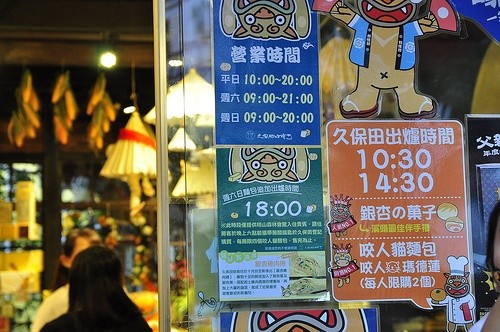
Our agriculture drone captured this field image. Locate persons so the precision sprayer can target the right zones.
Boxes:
[466,202,500,331]
[30,227,129,332]
[38,246,153,332]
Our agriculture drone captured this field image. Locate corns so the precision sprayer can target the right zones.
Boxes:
[7,70,116,150]
